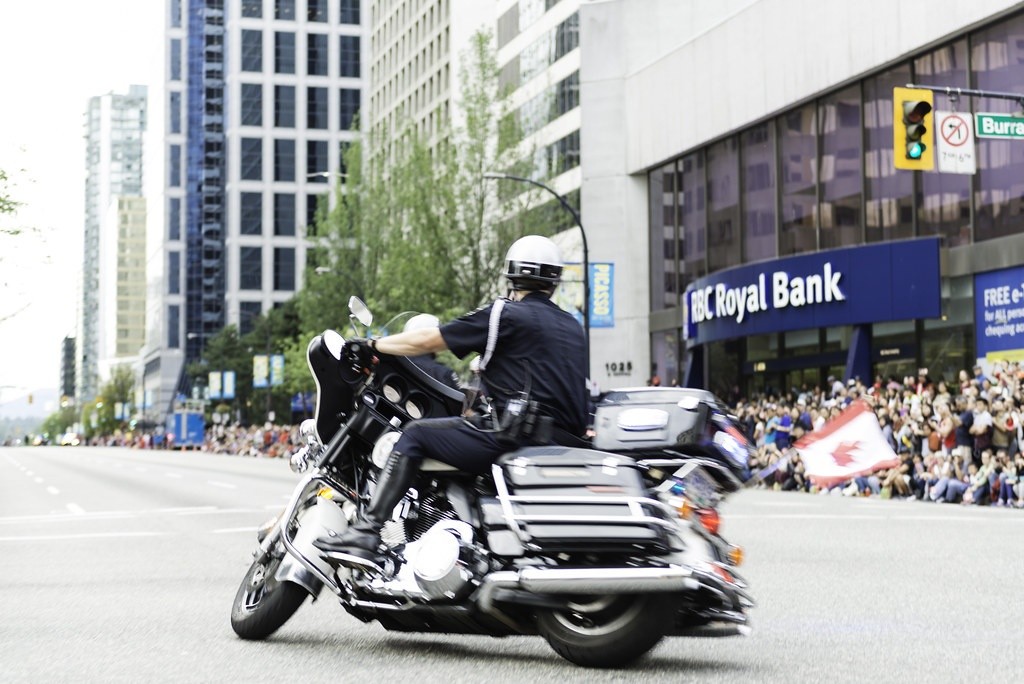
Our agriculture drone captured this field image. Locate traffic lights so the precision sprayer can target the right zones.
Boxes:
[893,86,935,170]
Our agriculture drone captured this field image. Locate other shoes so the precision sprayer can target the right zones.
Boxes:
[830,486,841,495]
[907,495,1024,509]
[819,487,830,495]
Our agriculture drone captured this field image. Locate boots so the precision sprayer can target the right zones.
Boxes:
[312,443,424,560]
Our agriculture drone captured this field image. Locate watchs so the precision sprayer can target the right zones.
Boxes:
[370,335,384,355]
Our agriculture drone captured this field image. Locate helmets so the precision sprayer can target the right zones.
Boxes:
[501,234,565,286]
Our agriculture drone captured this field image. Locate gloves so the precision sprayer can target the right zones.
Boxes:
[340,337,375,377]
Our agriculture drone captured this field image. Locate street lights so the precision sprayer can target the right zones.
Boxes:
[484,168,590,383]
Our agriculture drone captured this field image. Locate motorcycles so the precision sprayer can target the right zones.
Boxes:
[229,296,759,671]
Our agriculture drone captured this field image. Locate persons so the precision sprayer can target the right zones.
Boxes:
[650,376,662,386]
[312,234,590,561]
[672,378,681,387]
[714,359,1024,510]
[90,421,301,458]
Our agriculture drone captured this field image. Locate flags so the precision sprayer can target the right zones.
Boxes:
[791,398,900,492]
